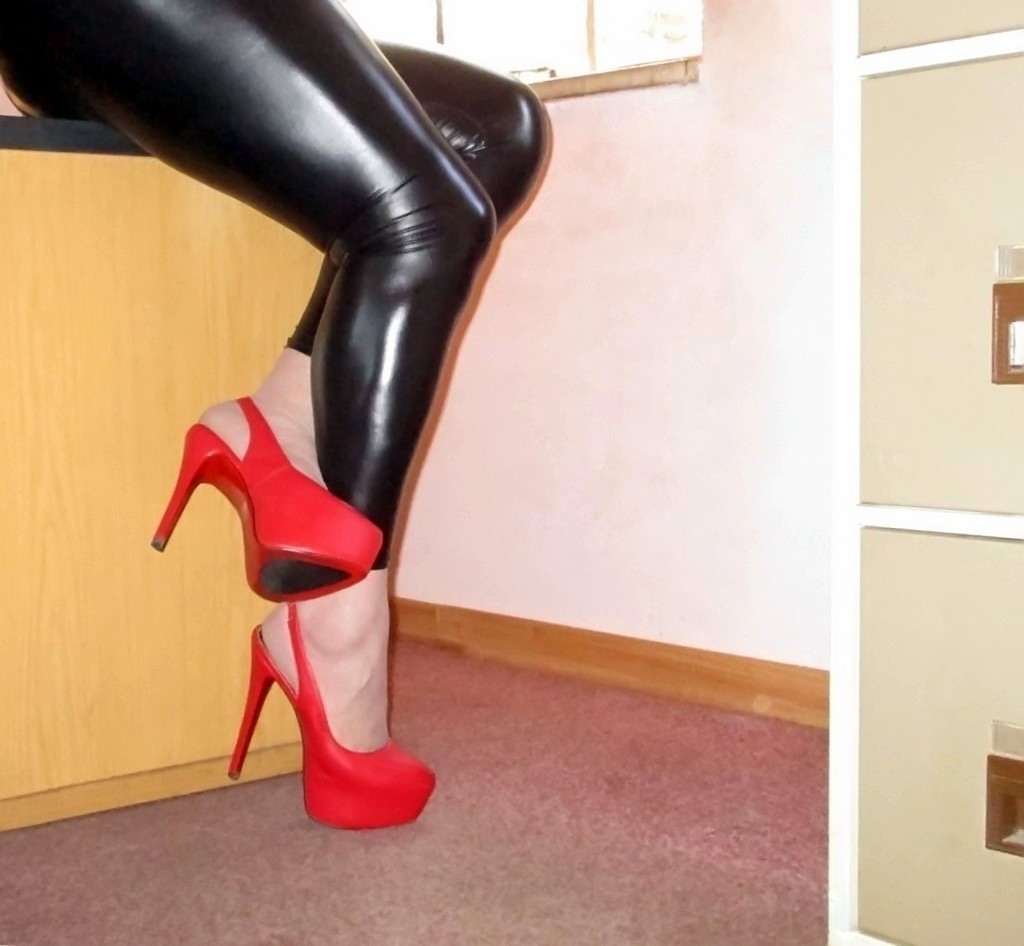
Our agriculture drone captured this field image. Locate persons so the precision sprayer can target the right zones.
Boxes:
[0,0,554,829]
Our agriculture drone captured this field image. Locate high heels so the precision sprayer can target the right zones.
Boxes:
[227,603,438,829]
[151,397,382,603]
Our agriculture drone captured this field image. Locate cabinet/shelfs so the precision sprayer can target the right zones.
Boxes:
[0,115,324,831]
